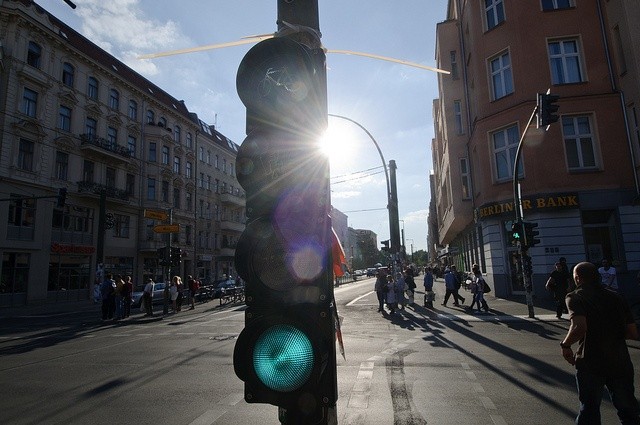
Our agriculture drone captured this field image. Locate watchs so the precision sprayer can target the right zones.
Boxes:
[561,341,571,349]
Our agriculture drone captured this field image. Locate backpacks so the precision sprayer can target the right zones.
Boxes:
[120,284,128,297]
[193,280,200,290]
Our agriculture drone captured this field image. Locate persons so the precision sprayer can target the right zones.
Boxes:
[561,262,640,418]
[100,273,133,320]
[599,259,618,289]
[545,262,567,320]
[451,265,461,306]
[143,278,155,317]
[473,264,482,279]
[474,271,490,314]
[558,257,570,314]
[170,276,183,314]
[187,275,199,310]
[466,268,483,314]
[219,273,242,289]
[441,269,465,305]
[373,263,417,315]
[423,267,434,309]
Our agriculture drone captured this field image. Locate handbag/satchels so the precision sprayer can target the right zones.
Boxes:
[483,279,491,292]
[403,283,409,292]
[143,283,153,297]
[425,289,436,302]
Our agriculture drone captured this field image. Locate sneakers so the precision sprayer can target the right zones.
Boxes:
[462,298,465,304]
[453,302,455,304]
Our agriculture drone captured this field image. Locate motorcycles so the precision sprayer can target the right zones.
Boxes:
[380,267,388,278]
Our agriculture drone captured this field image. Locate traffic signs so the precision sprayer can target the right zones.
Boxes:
[144,209,169,221]
[152,225,180,233]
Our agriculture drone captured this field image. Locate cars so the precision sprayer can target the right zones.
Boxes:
[130,283,179,308]
[363,270,368,274]
[356,270,362,275]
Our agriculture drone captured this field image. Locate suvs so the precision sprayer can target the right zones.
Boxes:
[367,268,376,277]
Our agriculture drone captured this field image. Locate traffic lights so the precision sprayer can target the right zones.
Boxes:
[172,248,181,265]
[512,223,523,242]
[525,223,540,248]
[57,188,68,207]
[537,92,560,130]
[233,26,333,422]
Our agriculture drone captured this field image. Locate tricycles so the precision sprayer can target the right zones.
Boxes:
[220,286,244,305]
